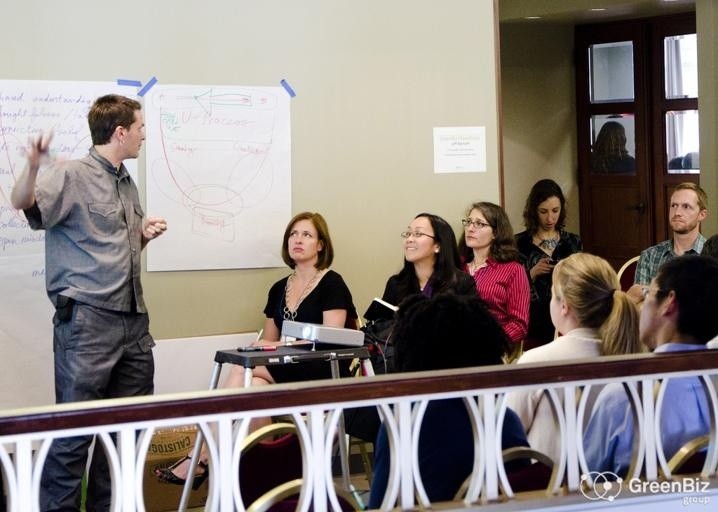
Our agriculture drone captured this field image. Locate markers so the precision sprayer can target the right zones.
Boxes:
[237,345,277,352]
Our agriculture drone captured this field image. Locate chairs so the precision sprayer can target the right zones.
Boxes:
[451,446,570,503]
[251,306,375,487]
[234,421,305,508]
[240,477,361,512]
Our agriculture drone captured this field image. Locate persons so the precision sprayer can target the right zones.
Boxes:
[359,178,718,512]
[594,120,637,173]
[151,213,359,490]
[11,95,169,511]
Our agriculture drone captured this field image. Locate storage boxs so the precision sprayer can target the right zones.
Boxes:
[134,424,210,512]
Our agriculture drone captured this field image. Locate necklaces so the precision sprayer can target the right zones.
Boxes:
[283,268,323,319]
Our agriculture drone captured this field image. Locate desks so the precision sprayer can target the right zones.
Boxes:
[175,342,391,512]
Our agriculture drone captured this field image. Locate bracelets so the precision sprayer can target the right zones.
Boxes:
[285,340,293,347]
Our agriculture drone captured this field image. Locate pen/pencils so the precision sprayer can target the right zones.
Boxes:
[256,328,264,341]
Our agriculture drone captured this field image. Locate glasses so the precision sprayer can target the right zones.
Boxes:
[461,219,490,229]
[400,231,434,240]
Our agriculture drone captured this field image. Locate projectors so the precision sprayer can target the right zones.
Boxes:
[282,320,365,351]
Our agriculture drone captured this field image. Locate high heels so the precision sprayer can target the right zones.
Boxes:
[154,456,208,490]
